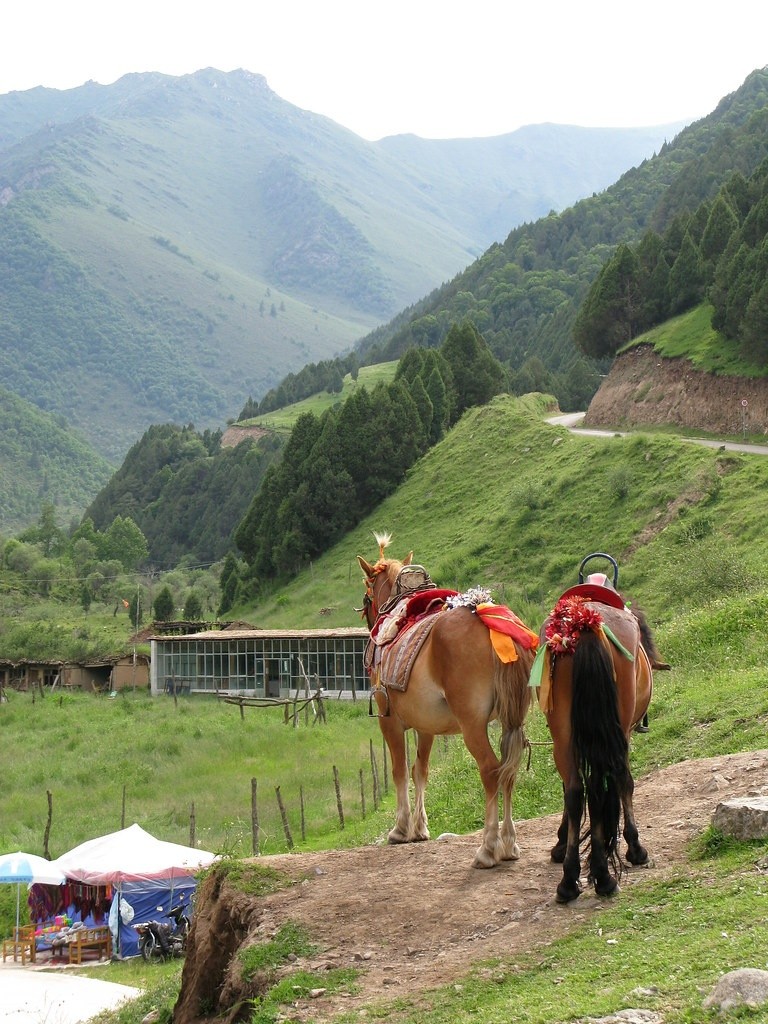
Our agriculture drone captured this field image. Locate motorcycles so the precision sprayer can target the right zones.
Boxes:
[130,893,192,967]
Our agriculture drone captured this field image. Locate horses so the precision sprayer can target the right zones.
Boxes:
[355,531,541,871]
[536,571,671,905]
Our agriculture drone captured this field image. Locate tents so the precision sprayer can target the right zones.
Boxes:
[36,821,229,961]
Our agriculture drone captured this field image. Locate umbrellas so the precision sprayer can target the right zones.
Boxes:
[0,850,67,943]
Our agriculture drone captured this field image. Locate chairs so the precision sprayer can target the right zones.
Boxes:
[2,926,36,965]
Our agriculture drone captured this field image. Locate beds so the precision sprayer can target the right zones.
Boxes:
[21,919,110,965]
